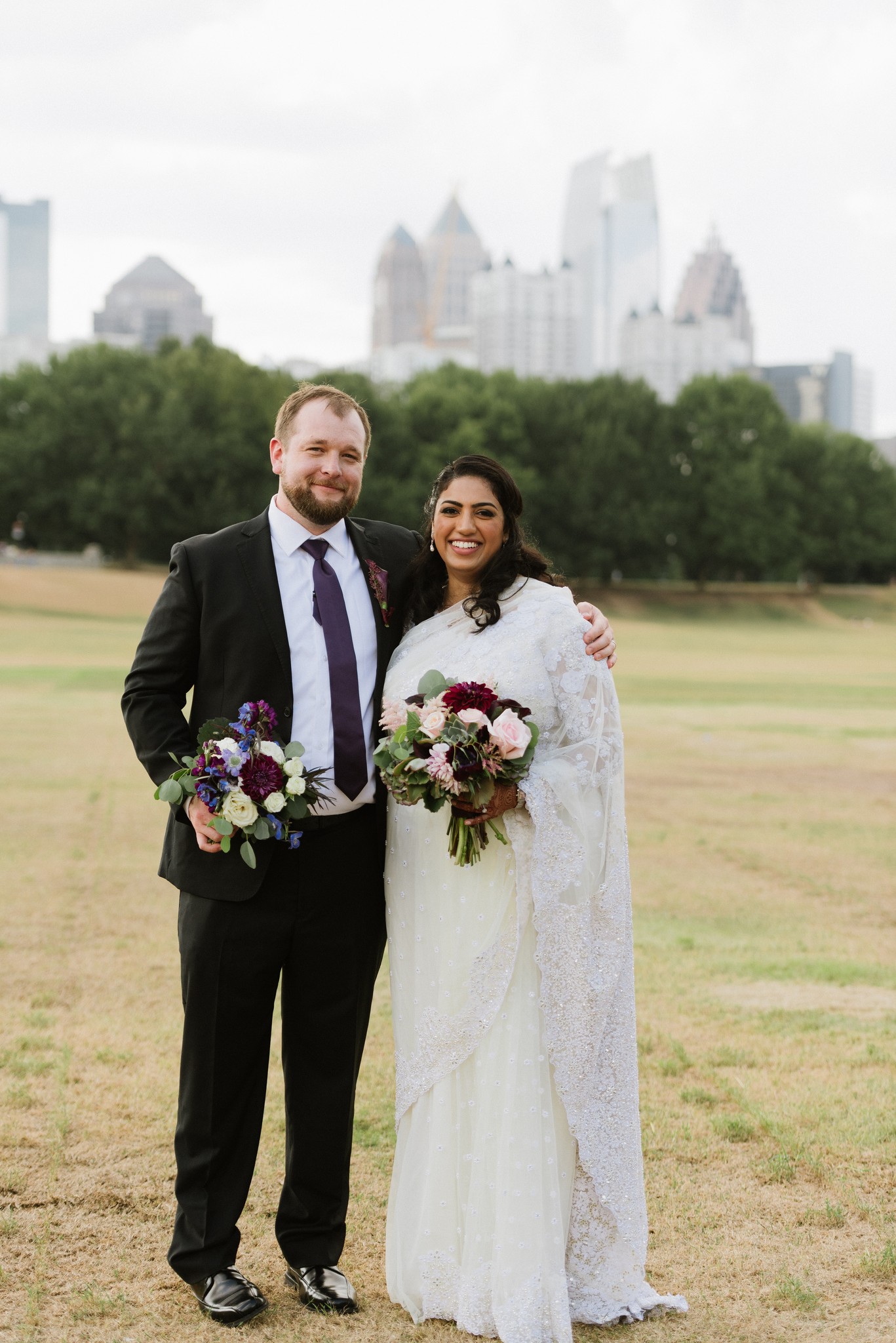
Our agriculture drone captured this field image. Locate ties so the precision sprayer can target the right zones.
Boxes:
[301,538,367,800]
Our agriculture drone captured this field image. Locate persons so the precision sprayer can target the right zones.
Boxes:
[118,383,615,1325]
[375,454,691,1343]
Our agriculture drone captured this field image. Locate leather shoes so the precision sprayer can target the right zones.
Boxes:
[190,1265,268,1327]
[284,1264,359,1313]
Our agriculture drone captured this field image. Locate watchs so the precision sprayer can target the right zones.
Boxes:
[511,784,526,809]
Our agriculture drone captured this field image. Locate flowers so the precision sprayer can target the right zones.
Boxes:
[364,559,395,627]
[373,669,539,867]
[154,700,337,869]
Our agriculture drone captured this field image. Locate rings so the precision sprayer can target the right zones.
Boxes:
[482,804,487,813]
